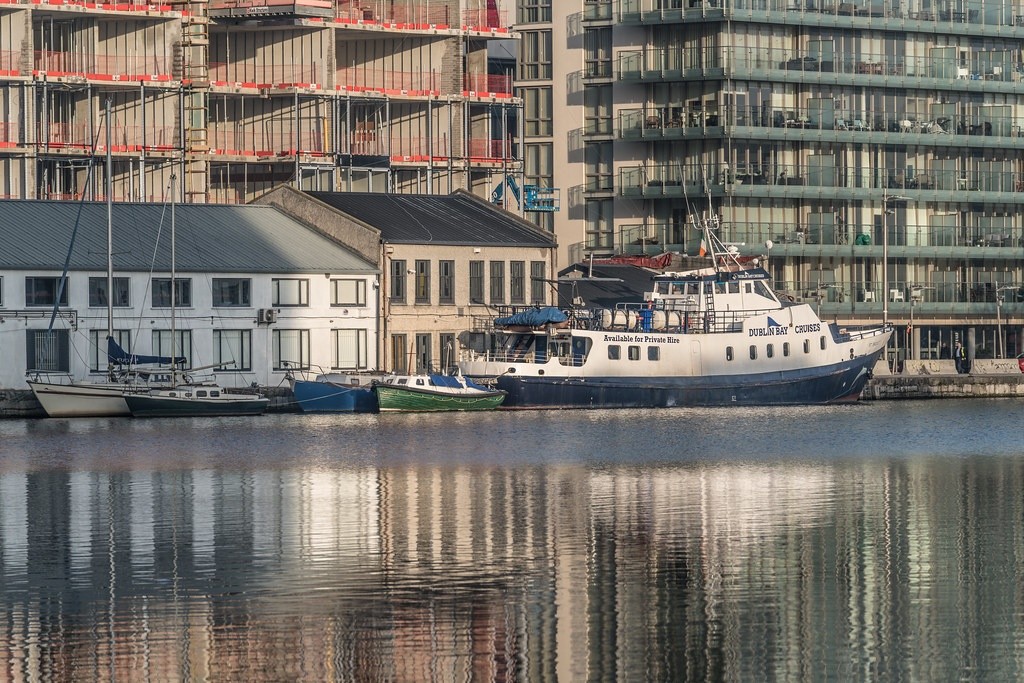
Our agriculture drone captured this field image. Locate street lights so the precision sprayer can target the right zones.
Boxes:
[996,280,1020,359]
[910,280,935,359]
[883,187,913,359]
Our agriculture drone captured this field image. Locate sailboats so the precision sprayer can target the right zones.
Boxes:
[24,95,271,417]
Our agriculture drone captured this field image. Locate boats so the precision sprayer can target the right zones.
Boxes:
[280,360,386,411]
[376,374,509,413]
[458,210,895,408]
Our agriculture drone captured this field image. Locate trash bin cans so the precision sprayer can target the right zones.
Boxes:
[638,308,652,332]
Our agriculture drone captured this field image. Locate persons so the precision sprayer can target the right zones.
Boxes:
[940,343,950,359]
[954,343,967,374]
[640,300,653,332]
